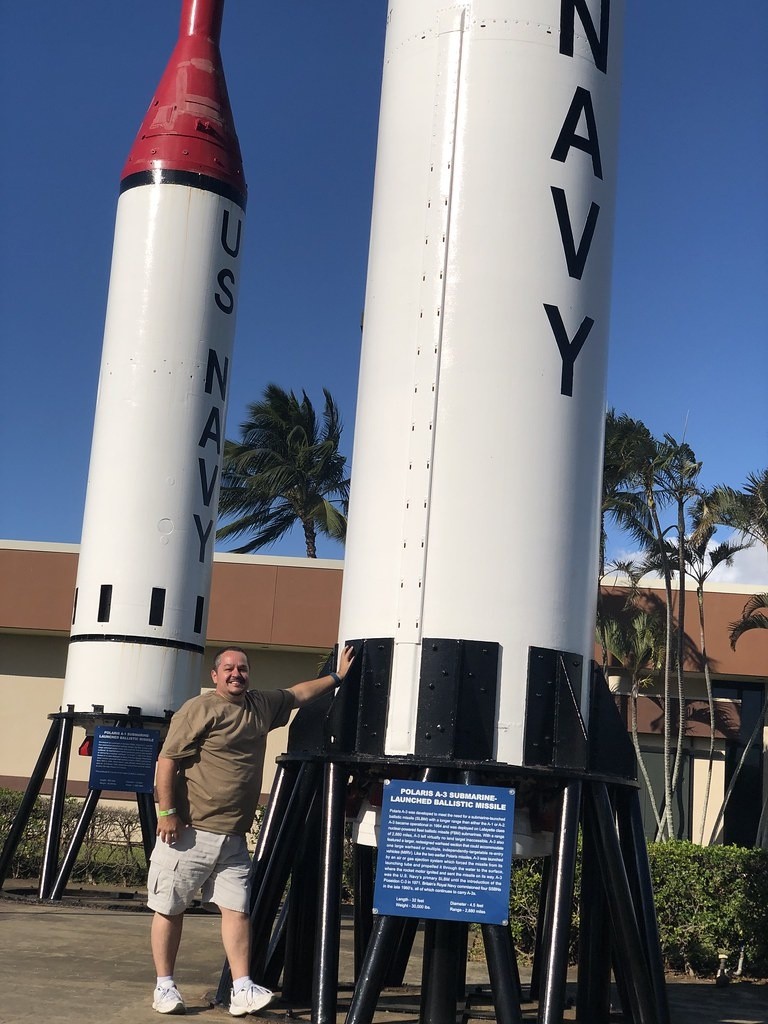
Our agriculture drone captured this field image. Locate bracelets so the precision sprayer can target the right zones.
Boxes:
[158,807,177,817]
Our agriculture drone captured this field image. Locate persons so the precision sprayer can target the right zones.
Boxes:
[144,646,358,1016]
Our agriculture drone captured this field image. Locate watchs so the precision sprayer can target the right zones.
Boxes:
[330,671,342,688]
